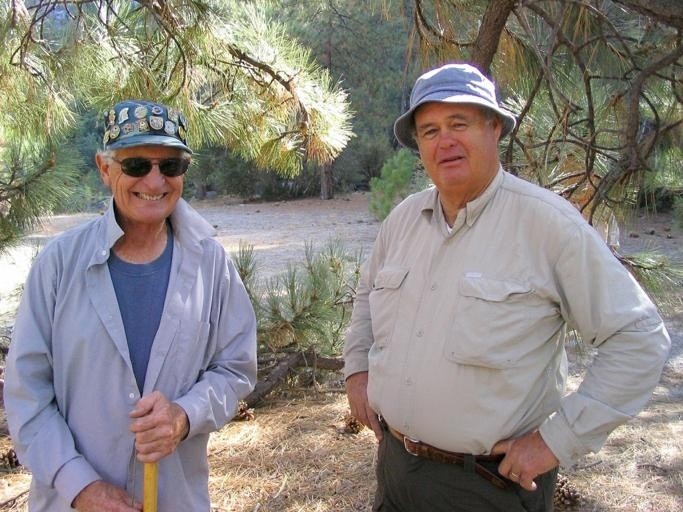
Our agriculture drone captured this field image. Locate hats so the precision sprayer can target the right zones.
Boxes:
[101,100,195,156]
[393,62,517,153]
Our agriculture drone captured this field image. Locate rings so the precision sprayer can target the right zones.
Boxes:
[509,470,521,479]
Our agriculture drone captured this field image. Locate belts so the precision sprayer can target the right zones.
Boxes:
[385,425,508,490]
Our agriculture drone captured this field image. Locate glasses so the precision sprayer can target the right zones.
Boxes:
[113,157,191,178]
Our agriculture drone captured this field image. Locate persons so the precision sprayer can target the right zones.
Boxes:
[338,63,670,512]
[0,94,258,511]
